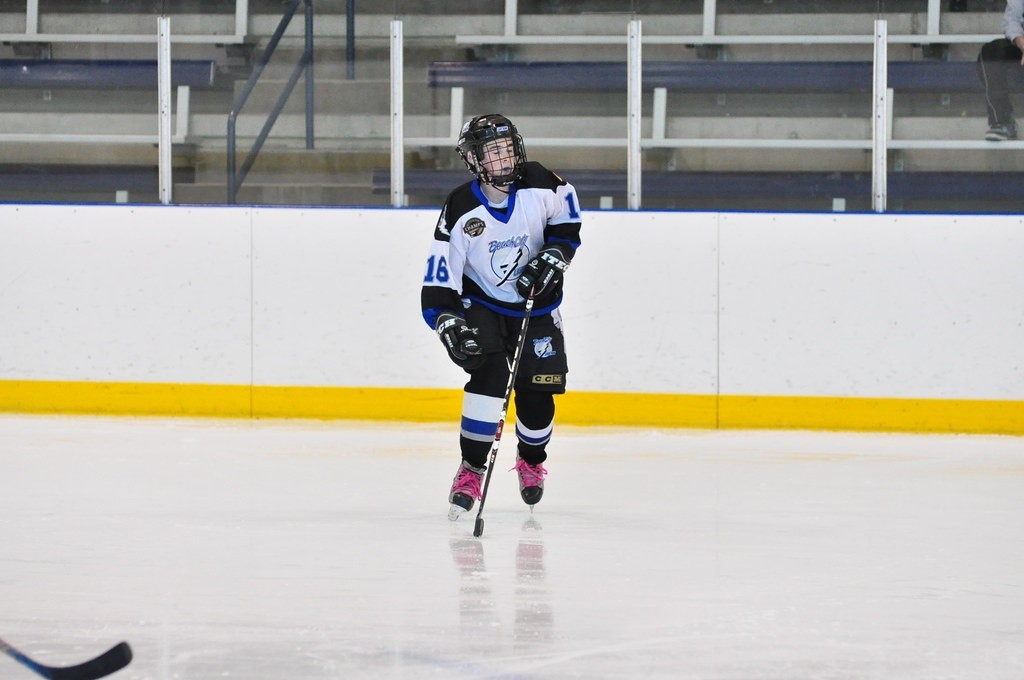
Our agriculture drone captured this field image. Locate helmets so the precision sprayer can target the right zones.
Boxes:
[455,114,523,186]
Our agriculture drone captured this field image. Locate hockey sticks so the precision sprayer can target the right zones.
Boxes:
[0,638,133,680]
[474,290,539,535]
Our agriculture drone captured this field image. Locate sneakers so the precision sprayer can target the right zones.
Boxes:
[448,458,487,521]
[508,448,548,513]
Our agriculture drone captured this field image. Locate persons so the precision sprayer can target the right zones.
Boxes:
[421,116,582,523]
[978,0,1024,141]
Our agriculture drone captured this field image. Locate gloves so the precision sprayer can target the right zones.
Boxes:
[435,311,487,373]
[516,241,576,300]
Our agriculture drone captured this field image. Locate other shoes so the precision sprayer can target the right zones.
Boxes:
[984,119,1018,141]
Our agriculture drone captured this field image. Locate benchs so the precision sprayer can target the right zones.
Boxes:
[0,0,257,205]
[372,0,1024,214]
